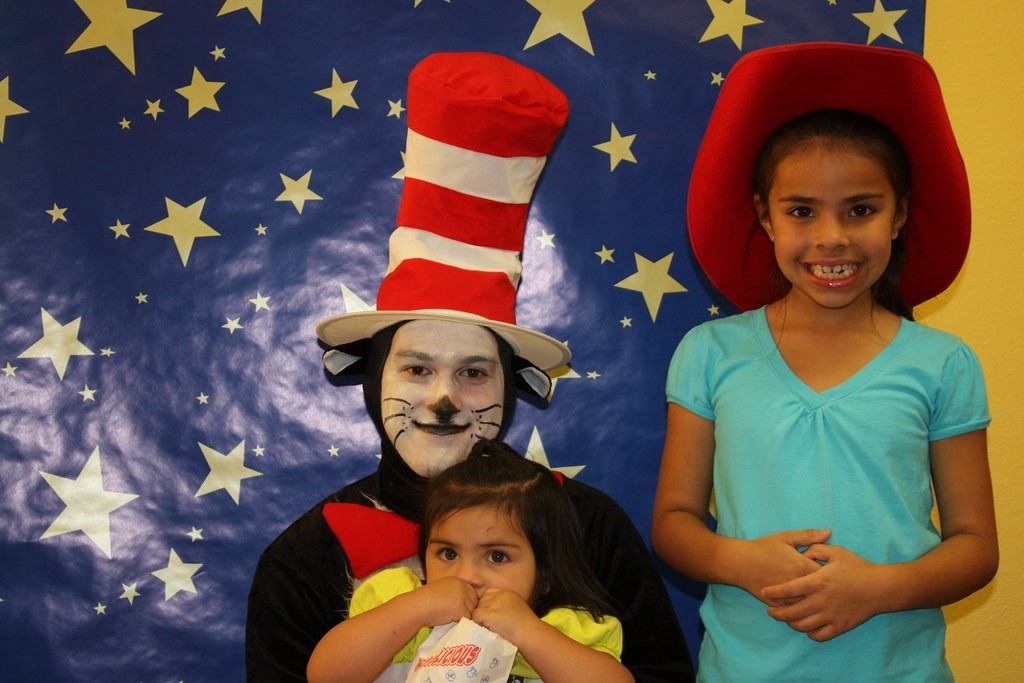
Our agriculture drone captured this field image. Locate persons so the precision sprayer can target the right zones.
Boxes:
[311,450,636,683]
[248,49,696,681]
[649,44,999,683]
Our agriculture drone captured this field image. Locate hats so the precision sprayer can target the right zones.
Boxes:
[309,48,574,373]
[685,40,971,313]
